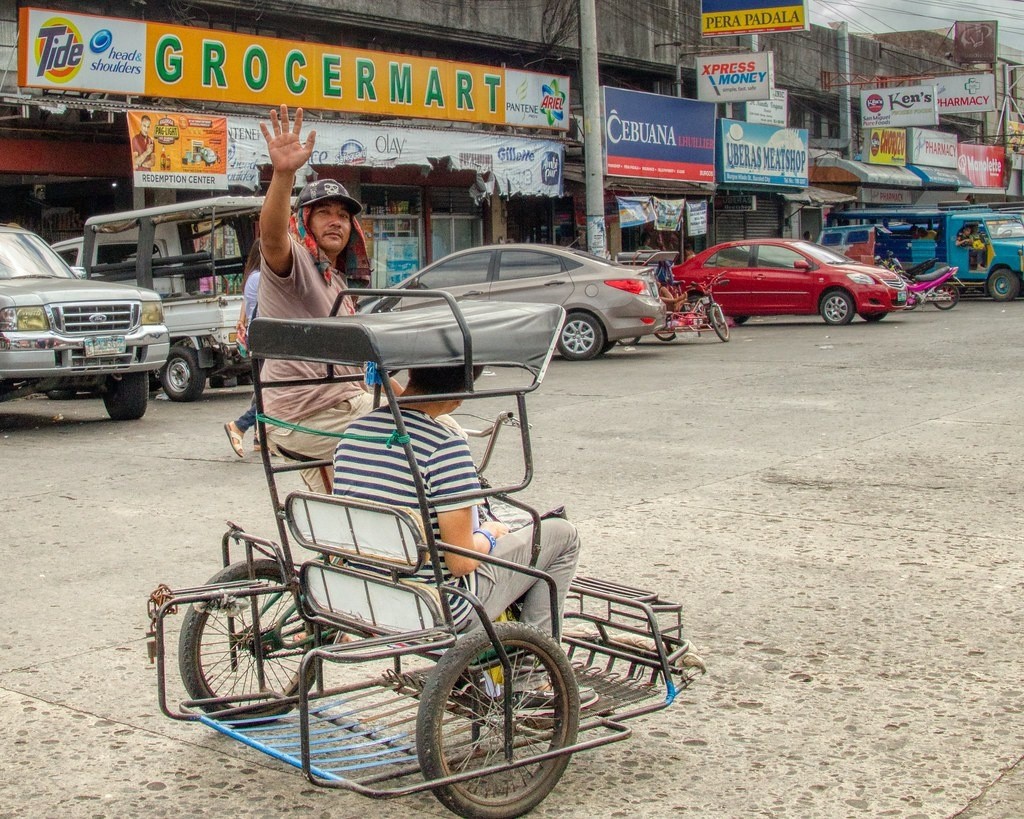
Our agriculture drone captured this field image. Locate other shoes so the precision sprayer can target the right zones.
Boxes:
[976,267,987,271]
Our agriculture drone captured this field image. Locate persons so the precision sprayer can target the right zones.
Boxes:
[803,231,812,241]
[1008,125,1024,154]
[634,233,652,251]
[258,103,405,648]
[196,249,218,293]
[333,364,599,716]
[657,278,687,313]
[132,115,155,172]
[684,243,696,262]
[909,226,937,240]
[956,224,987,271]
[224,235,271,457]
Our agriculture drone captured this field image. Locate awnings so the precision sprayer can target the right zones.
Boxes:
[907,164,973,188]
[778,185,857,204]
[808,158,923,187]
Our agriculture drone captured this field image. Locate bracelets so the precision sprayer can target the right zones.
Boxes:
[473,529,496,555]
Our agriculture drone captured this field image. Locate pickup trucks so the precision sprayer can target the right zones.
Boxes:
[49,194,303,402]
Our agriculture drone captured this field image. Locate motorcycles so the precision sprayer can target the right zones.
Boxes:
[874,250,965,310]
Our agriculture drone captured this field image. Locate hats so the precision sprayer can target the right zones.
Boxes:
[298,178,363,217]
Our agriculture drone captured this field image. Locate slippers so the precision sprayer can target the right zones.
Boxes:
[224,422,245,458]
[254,445,271,451]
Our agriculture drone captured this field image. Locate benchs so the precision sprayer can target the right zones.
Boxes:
[285,492,562,673]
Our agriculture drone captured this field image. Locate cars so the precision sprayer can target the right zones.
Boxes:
[354,242,667,361]
[0,221,169,419]
[671,238,908,326]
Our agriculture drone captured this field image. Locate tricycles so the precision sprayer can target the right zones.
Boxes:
[145,290,705,818]
[617,270,729,346]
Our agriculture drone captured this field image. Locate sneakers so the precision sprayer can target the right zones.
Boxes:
[511,655,547,673]
[512,686,599,717]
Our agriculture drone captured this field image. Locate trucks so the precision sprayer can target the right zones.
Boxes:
[815,203,1023,302]
